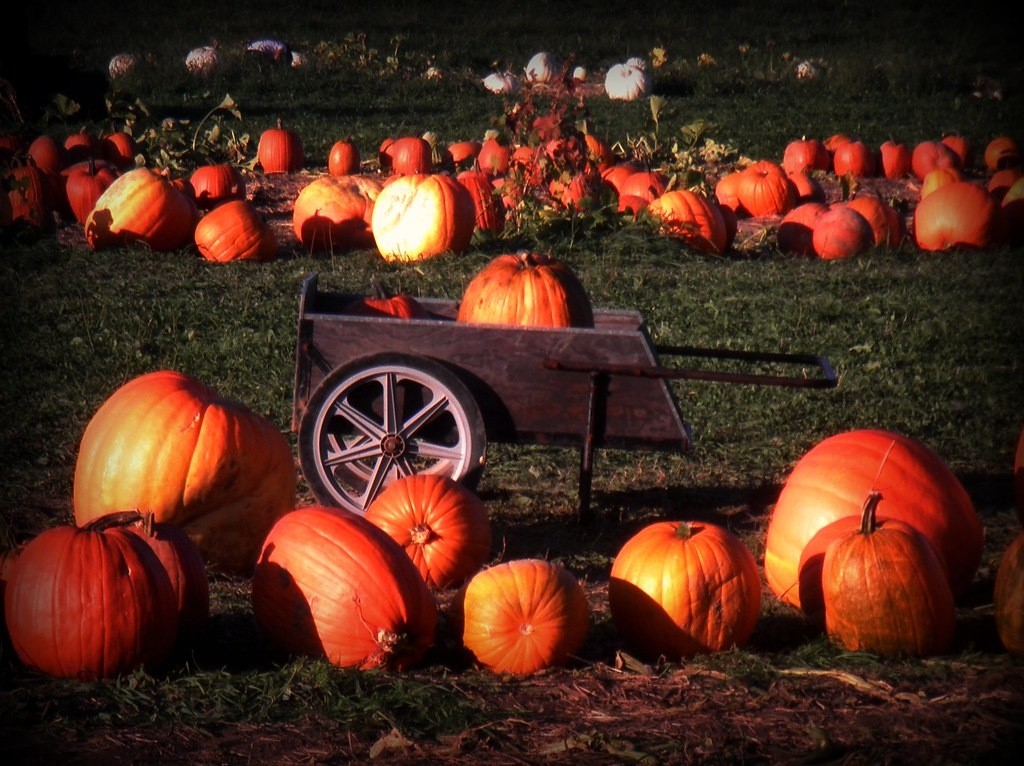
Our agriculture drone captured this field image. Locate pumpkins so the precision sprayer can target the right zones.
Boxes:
[253,505,435,673]
[366,475,492,591]
[2,33,1022,332]
[762,424,984,662]
[72,373,296,571]
[607,521,759,659]
[3,512,212,680]
[447,558,589,677]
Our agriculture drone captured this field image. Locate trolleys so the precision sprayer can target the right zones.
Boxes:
[287,276,845,535]
